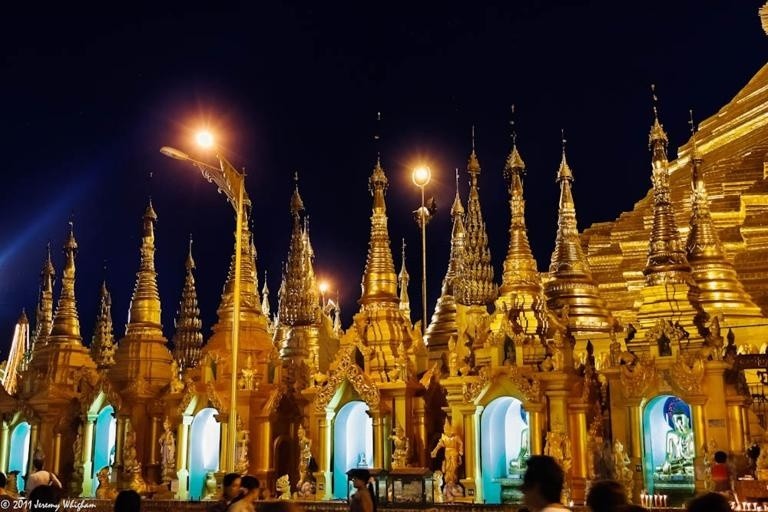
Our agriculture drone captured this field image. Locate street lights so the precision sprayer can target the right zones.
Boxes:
[161,129,246,473]
[413,164,431,332]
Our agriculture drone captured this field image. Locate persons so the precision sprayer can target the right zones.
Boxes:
[158,416,176,487]
[295,424,313,489]
[205,473,242,512]
[240,361,258,391]
[430,415,463,487]
[224,475,260,512]
[502,344,515,366]
[111,490,141,511]
[122,421,136,472]
[662,417,693,474]
[610,436,634,483]
[0,472,24,512]
[712,450,731,500]
[24,458,62,512]
[397,343,407,384]
[586,476,628,512]
[234,414,251,477]
[348,469,373,512]
[686,492,732,512]
[387,422,409,471]
[447,335,457,377]
[71,433,82,470]
[542,417,572,473]
[518,455,572,512]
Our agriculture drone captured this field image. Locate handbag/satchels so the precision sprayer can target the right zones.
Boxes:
[50,480,63,505]
